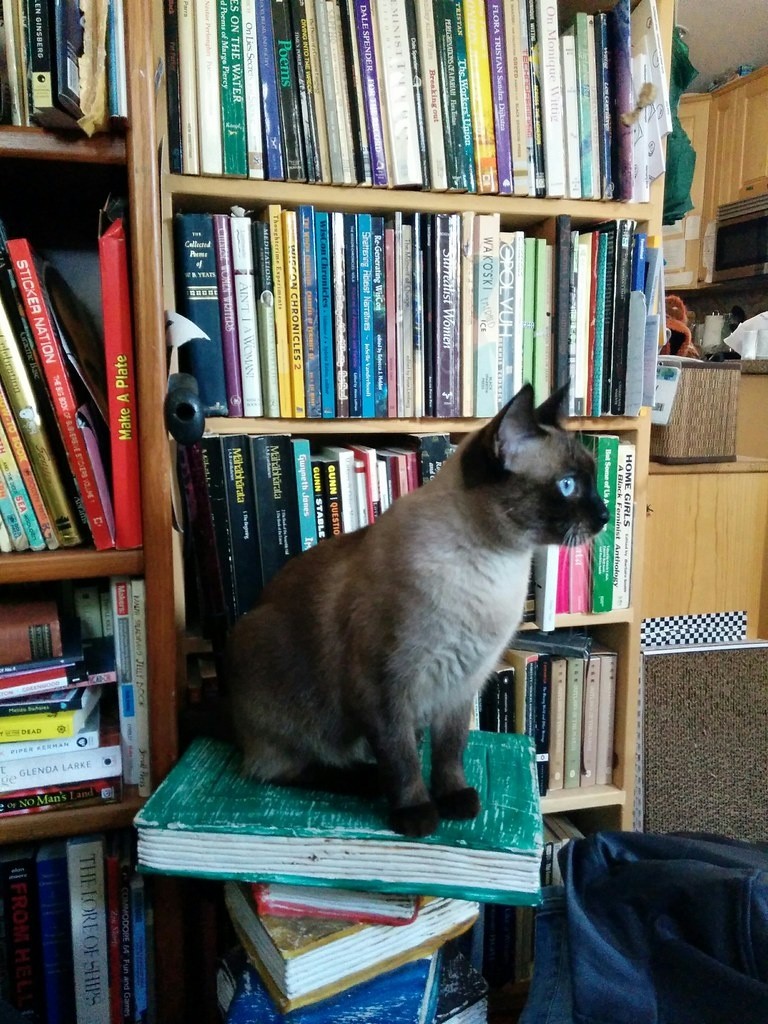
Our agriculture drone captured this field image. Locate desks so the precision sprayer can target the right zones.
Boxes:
[647,455,768,640]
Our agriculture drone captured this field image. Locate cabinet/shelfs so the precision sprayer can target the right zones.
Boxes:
[152,0,677,1024]
[0,0,162,1024]
[715,71,768,206]
[662,92,713,291]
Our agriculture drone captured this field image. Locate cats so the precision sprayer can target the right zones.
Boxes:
[184,379,611,838]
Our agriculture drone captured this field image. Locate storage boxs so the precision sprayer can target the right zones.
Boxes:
[649,358,742,466]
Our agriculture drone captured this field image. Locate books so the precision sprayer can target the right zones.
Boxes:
[0,0,127,140]
[178,1,648,802]
[111,727,557,1019]
[1,213,147,1023]
